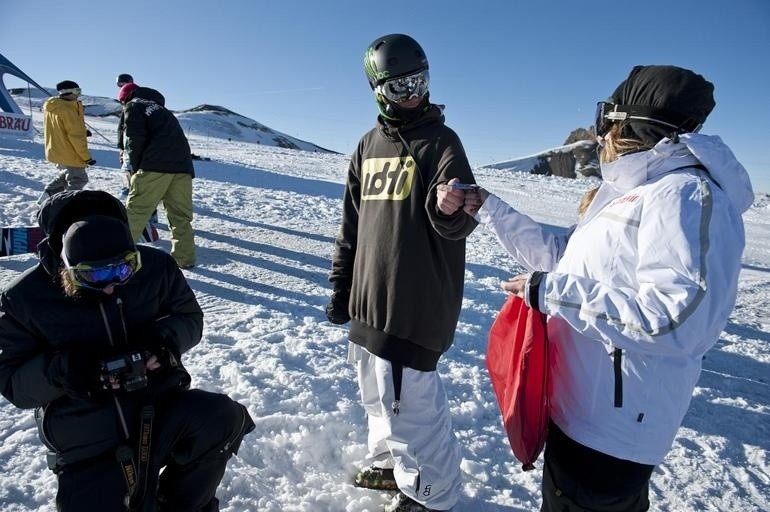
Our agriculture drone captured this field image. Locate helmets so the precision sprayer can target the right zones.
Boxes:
[117,74,138,102]
[365,34,429,87]
[65,216,136,266]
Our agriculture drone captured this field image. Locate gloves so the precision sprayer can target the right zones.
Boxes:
[85,159,96,165]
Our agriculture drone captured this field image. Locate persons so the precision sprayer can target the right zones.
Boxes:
[465,65,754,512]
[37,80,96,205]
[327,34,477,512]
[117,83,196,270]
[116,74,155,224]
[0,189,252,510]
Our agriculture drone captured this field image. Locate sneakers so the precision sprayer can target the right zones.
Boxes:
[356,468,398,489]
[385,492,437,512]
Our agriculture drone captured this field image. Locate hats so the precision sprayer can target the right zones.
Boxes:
[57,80,79,95]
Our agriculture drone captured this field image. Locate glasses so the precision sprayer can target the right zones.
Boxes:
[382,69,428,102]
[59,88,81,96]
[595,101,677,137]
[73,252,141,291]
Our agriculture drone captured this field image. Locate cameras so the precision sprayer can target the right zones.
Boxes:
[104,350,152,393]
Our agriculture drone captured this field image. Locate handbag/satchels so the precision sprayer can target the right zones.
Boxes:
[488,293,548,471]
